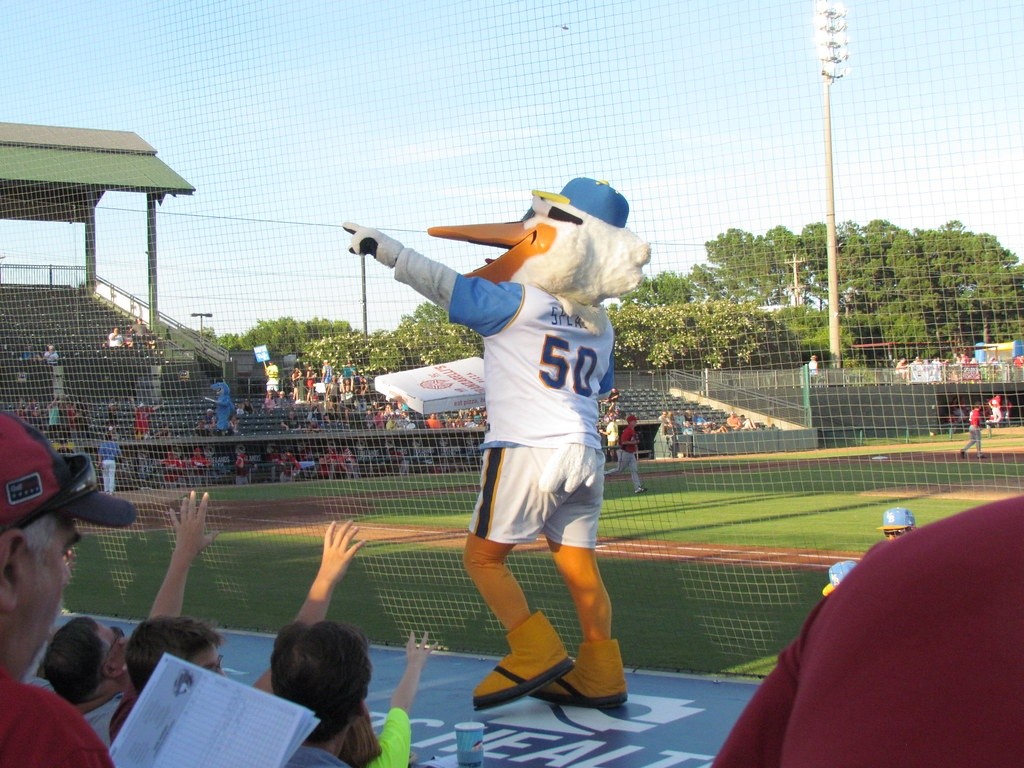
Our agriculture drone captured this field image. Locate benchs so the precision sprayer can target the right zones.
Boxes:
[633,448,653,459]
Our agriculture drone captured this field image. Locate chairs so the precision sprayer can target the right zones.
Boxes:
[606,387,782,435]
[0,289,460,440]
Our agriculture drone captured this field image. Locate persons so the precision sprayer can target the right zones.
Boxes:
[710,493,1024,768]
[19,345,59,365]
[271,622,372,767]
[948,395,1011,432]
[658,410,728,435]
[895,353,1024,384]
[604,416,647,493]
[876,507,916,541]
[108,318,147,348]
[822,561,856,596]
[18,361,488,496]
[1,408,138,768]
[809,355,818,388]
[338,631,438,768]
[727,411,758,431]
[110,491,366,744]
[960,402,986,458]
[599,388,621,457]
[44,617,129,748]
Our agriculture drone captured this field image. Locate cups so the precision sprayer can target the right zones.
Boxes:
[454,721,485,768]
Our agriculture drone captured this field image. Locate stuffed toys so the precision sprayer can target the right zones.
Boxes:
[342,177,652,711]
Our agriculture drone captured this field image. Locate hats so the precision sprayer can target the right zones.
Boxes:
[627,415,638,423]
[0,411,138,537]
[822,561,858,597]
[877,509,915,532]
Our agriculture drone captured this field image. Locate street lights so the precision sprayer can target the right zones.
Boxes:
[191,313,213,346]
[815,2,851,370]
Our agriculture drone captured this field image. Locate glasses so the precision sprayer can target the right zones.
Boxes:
[99,625,125,676]
[884,527,911,537]
[18,454,100,532]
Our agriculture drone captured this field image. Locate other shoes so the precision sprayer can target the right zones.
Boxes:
[635,486,646,494]
[961,450,965,459]
[978,456,989,462]
